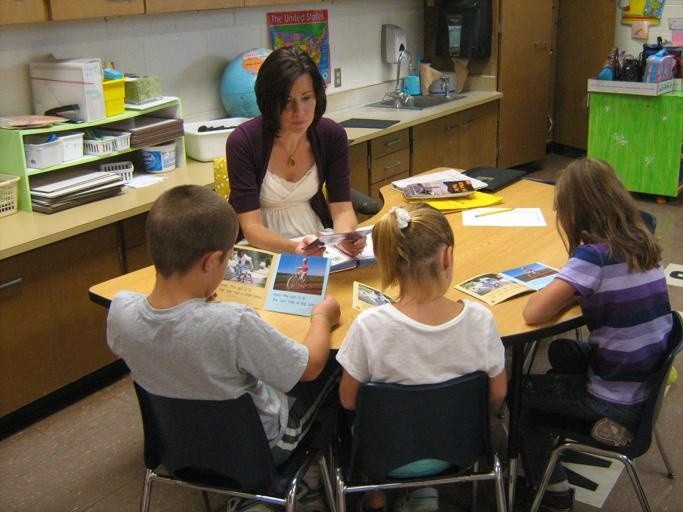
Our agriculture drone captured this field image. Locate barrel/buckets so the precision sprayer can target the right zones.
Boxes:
[142,141,176,174]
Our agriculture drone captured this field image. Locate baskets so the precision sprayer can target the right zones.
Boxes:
[84,128,132,157]
[0,174,21,217]
[100,161,134,184]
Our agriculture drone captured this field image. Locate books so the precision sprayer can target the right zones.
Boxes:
[390,167,489,200]
[288,224,377,274]
[461,165,526,194]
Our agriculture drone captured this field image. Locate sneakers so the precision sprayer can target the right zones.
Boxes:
[224,480,329,512]
[510,474,577,512]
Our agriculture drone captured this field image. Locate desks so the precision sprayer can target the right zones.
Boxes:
[87,168,634,512]
[587,89,682,205]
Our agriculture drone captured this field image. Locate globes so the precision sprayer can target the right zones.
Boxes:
[220,47,273,117]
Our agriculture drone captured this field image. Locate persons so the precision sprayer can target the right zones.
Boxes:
[224,46,370,260]
[332,198,510,511]
[105,184,344,512]
[496,157,672,511]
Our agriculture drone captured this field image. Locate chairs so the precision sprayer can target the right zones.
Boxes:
[527,310,683,511]
[333,379,515,512]
[348,180,383,221]
[631,207,657,237]
[130,381,334,512]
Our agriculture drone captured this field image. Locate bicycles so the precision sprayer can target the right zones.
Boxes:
[286,267,310,291]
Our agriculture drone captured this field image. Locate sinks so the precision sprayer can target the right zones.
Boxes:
[374,92,467,111]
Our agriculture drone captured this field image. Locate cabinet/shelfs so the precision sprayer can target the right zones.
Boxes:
[371,129,408,204]
[424,1,618,170]
[1,217,121,423]
[413,102,500,181]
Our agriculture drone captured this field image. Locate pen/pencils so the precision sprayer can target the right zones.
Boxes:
[475,207,514,217]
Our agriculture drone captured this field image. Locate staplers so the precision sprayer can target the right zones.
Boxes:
[45,104,83,123]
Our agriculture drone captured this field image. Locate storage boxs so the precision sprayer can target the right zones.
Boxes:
[22,129,85,170]
[184,116,255,163]
[587,78,683,96]
[27,57,163,123]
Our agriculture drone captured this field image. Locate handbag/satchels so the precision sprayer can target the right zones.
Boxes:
[601,36,683,82]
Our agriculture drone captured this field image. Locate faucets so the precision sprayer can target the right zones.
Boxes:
[440,74,450,100]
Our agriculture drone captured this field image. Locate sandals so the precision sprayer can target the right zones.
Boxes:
[392,485,442,512]
